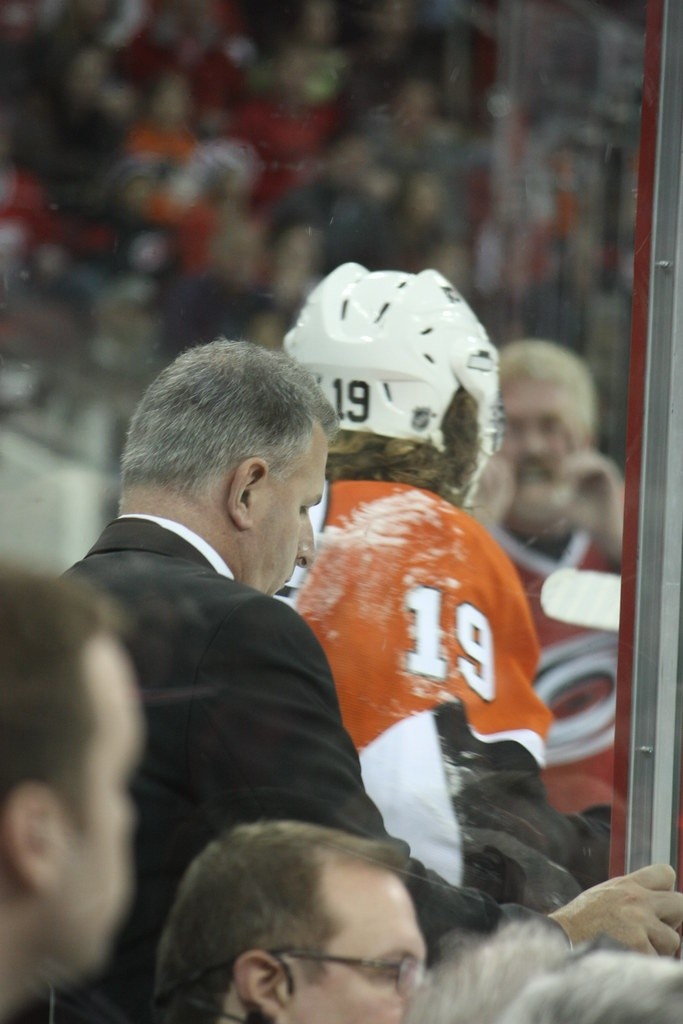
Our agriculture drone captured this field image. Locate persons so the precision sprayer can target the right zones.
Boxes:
[6,339,683,1024]
[149,822,424,1024]
[273,259,610,919]
[404,923,683,1024]
[0,0,644,427]
[466,341,625,815]
[0,562,142,1024]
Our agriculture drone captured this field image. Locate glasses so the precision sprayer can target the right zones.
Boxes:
[269,947,419,996]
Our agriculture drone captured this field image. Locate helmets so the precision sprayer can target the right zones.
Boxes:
[282,260,503,506]
